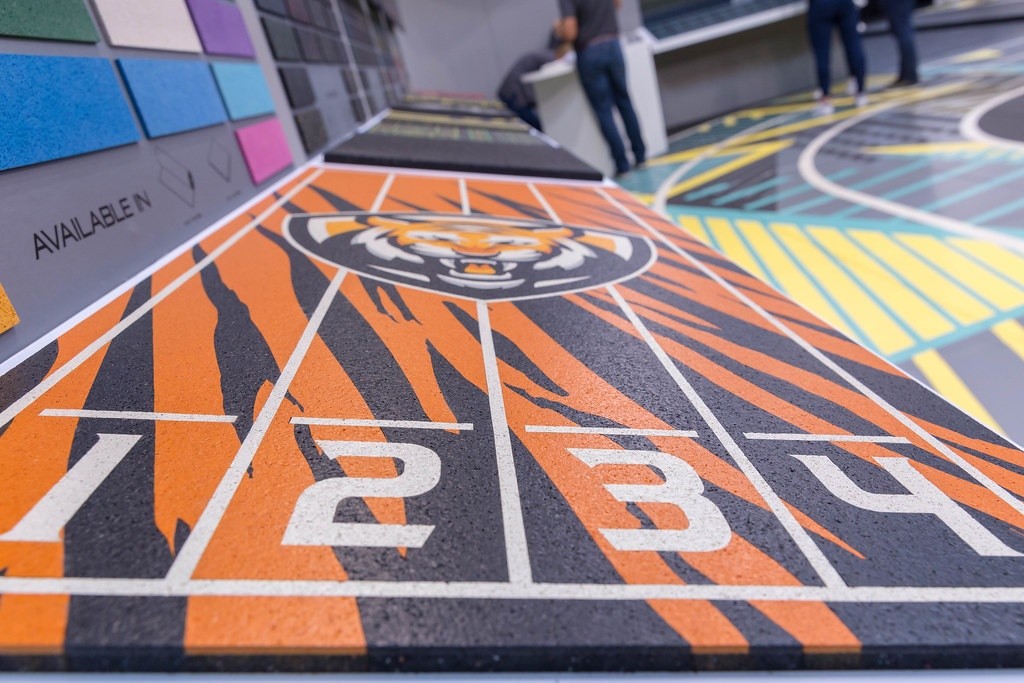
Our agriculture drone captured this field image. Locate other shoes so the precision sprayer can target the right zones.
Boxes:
[634,160,645,169]
[853,93,867,107]
[809,103,834,117]
[612,170,633,181]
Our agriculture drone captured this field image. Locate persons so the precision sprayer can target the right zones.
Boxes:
[552,0,646,177]
[873,1,934,91]
[806,0,868,118]
[499,33,570,132]
